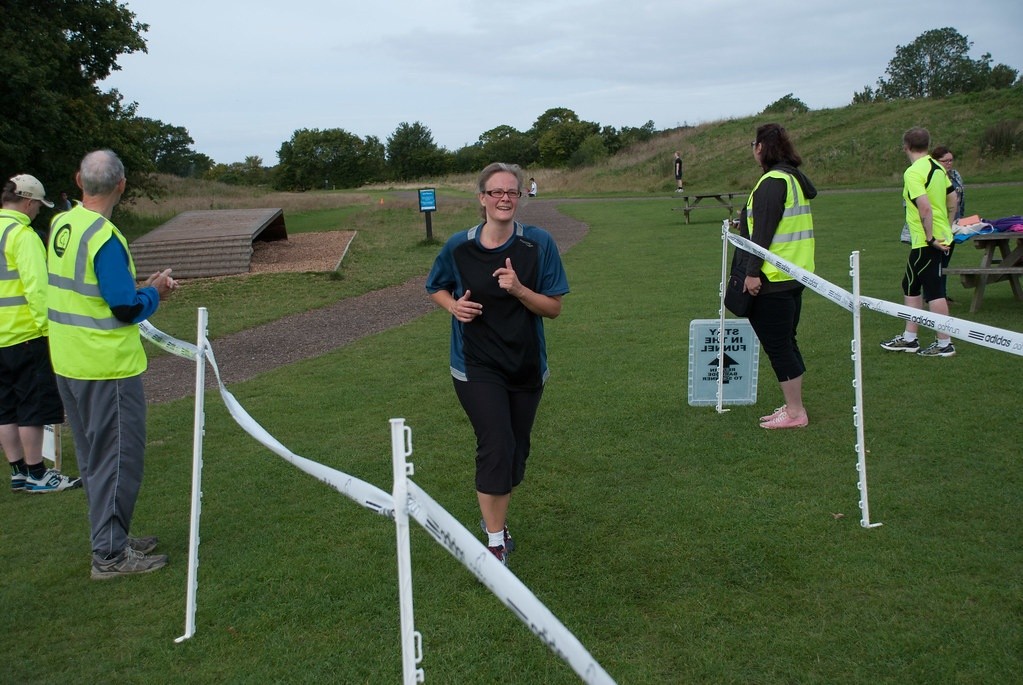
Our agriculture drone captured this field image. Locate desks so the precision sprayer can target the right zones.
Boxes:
[672,191,746,224]
[969,231,1023,314]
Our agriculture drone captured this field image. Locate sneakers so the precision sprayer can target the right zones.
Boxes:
[760,412,808,429]
[880,335,920,352]
[25,468,83,493]
[90,545,169,580]
[126,536,158,555]
[917,340,956,357]
[759,404,806,422]
[480,519,514,553]
[487,544,507,565]
[11,473,27,490]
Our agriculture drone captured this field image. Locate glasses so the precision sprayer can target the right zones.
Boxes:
[750,140,758,146]
[483,188,522,198]
[938,159,953,164]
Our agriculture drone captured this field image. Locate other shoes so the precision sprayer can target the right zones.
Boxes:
[925,296,955,304]
[675,188,683,193]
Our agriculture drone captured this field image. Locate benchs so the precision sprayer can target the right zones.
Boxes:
[940,259,1023,275]
[671,202,746,211]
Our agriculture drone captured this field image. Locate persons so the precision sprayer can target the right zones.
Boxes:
[59,190,72,210]
[49,150,177,579]
[424,163,568,564]
[730,121,818,429]
[932,146,965,304]
[674,151,683,192]
[527,178,538,197]
[0,167,84,494]
[879,126,956,358]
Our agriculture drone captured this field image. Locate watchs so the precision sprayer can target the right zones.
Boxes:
[925,236,936,245]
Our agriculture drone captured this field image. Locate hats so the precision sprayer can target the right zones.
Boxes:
[8,174,54,208]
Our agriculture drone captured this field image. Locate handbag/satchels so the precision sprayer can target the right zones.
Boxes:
[957,215,980,225]
[951,215,994,235]
[724,270,754,317]
